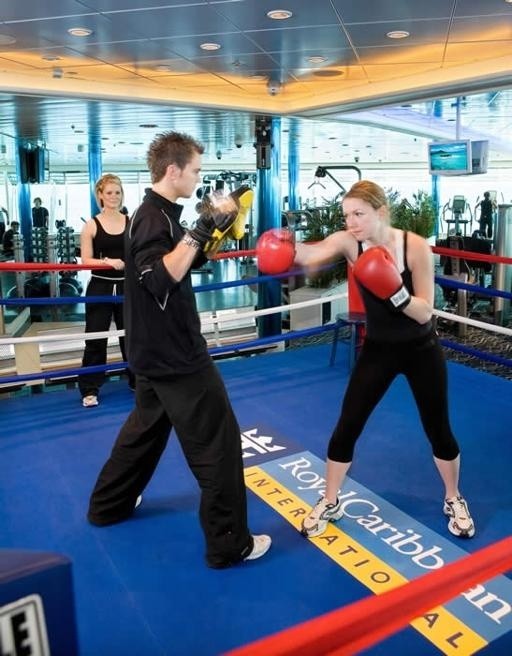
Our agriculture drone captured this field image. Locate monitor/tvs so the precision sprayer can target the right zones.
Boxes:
[19,145,30,184]
[471,140,489,175]
[427,139,473,175]
[33,146,45,183]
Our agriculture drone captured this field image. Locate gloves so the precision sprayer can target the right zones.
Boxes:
[352,246,412,314]
[255,228,298,274]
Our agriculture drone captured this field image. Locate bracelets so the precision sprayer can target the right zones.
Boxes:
[102,256,109,264]
[182,231,200,248]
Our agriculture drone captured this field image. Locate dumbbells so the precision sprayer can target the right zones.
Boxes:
[31,229,77,278]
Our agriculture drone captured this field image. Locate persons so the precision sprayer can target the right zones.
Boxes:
[88,129,273,569]
[78,171,134,408]
[31,197,49,233]
[2,220,20,256]
[0,204,10,244]
[475,191,498,239]
[252,179,479,542]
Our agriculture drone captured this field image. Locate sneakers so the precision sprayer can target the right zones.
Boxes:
[301,496,345,536]
[82,395,99,407]
[135,495,143,509]
[443,495,476,539]
[128,382,137,392]
[244,534,274,561]
